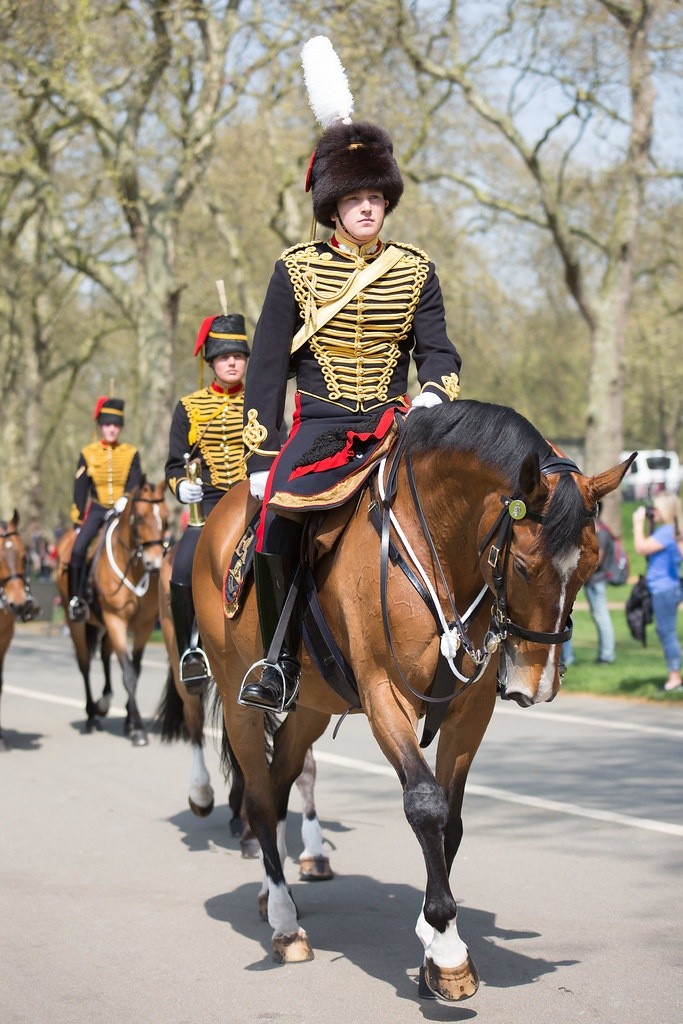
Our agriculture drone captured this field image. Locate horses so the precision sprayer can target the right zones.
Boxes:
[56,473,178,747]
[154,394,640,1002]
[0,508,41,700]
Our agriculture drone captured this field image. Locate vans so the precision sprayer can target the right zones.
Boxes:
[618,448,681,501]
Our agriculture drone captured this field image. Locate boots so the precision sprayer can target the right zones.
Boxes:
[170,581,211,694]
[240,544,309,712]
[68,563,86,617]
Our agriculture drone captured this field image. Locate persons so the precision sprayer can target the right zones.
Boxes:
[239,35,461,708]
[559,521,616,667]
[165,278,288,694]
[631,491,683,692]
[70,378,144,620]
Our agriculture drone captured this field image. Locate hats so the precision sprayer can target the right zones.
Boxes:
[300,36,404,230]
[193,280,250,363]
[93,378,125,427]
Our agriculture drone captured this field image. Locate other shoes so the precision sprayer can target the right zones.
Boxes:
[661,682,682,691]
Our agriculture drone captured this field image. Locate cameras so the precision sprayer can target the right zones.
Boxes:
[645,506,653,518]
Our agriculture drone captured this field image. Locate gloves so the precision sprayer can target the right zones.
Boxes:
[250,471,270,501]
[114,496,128,513]
[179,478,204,504]
[405,392,442,418]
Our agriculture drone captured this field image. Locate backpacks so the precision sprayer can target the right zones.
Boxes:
[594,522,630,586]
